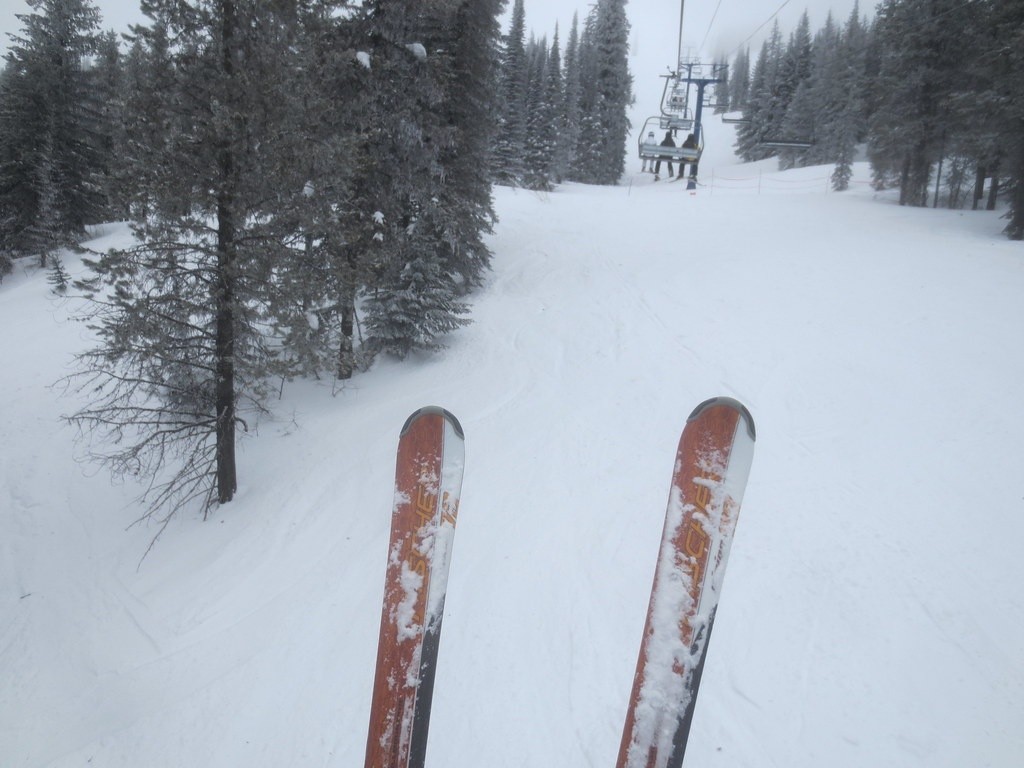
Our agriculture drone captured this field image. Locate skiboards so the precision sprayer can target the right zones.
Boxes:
[358,391,761,768]
[667,178,707,188]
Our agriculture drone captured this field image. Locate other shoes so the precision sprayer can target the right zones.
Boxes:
[676,175,683,179]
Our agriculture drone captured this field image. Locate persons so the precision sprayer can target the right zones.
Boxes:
[677,134,697,178]
[654,132,676,177]
[638,131,657,173]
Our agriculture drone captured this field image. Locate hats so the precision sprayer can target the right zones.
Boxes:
[649,132,655,136]
